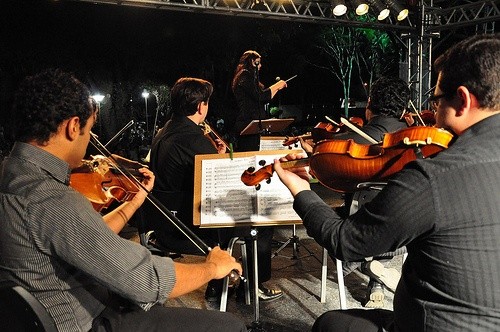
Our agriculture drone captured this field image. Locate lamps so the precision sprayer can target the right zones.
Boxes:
[329,0,409,23]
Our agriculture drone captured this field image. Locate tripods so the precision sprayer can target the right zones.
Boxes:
[270,224,329,271]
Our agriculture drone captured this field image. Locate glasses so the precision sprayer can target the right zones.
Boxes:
[426,89,455,108]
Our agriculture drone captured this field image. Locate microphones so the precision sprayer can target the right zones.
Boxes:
[276,77,280,83]
[216,119,225,131]
[253,61,259,67]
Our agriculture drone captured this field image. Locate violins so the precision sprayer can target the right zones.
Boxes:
[241,126,452,191]
[63,154,154,212]
[401,109,437,125]
[282,116,364,147]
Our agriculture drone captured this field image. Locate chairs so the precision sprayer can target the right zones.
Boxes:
[321,182,408,310]
[137,190,251,313]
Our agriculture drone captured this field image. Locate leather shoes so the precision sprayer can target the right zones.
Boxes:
[202,287,232,299]
[365,282,384,308]
[366,259,400,291]
[237,278,284,303]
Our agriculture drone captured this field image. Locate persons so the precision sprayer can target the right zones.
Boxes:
[300,75,412,307]
[230,50,288,147]
[273,33,500,332]
[0,67,249,332]
[150,77,283,303]
